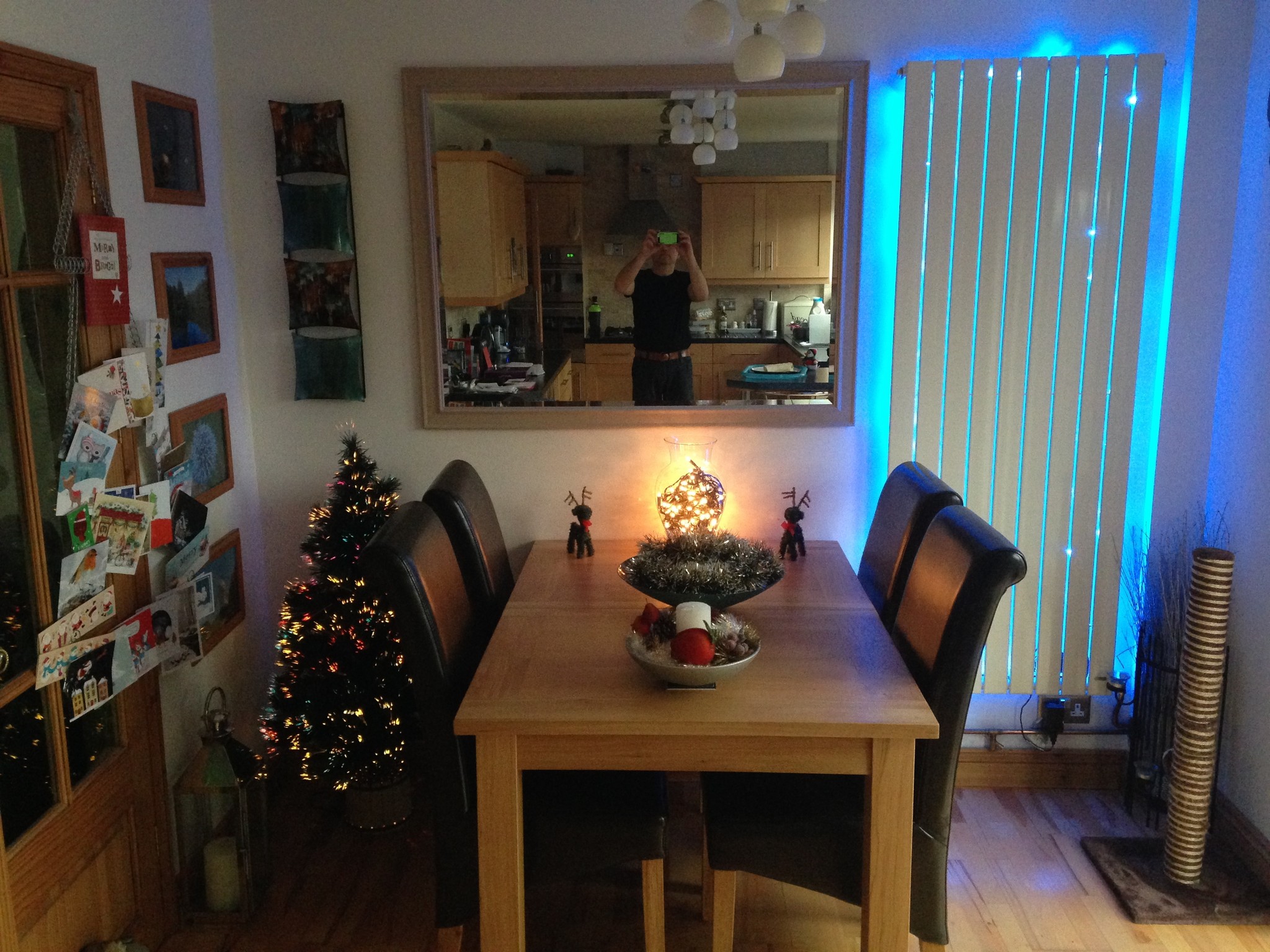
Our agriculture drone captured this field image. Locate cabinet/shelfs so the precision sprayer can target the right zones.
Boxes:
[699,183,832,284]
[547,343,801,400]
[438,161,583,305]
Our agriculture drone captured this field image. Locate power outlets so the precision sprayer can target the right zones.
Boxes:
[1037,696,1091,724]
[718,298,736,311]
[753,298,766,310]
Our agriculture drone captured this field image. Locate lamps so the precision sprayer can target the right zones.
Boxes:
[685,0,827,82]
[667,88,740,166]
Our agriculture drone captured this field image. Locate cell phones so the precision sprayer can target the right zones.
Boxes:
[657,232,679,245]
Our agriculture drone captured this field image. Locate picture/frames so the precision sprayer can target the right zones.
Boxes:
[131,80,207,206]
[191,528,245,666]
[150,250,223,366]
[169,392,237,504]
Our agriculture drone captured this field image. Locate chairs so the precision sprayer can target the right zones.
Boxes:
[422,459,514,633]
[363,501,670,952]
[706,505,1028,952]
[856,461,965,625]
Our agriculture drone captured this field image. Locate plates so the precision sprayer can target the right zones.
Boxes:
[751,366,801,375]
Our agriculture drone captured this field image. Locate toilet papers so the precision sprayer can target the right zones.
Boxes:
[762,300,777,331]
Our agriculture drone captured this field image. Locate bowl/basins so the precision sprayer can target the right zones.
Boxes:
[616,556,784,608]
[690,327,706,336]
[625,631,761,688]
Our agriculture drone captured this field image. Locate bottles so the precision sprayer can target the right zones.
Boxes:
[719,304,757,336]
[809,296,826,325]
[589,296,601,337]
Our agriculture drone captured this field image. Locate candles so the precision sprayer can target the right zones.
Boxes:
[204,836,238,909]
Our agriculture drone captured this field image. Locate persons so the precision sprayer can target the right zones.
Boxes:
[615,229,709,406]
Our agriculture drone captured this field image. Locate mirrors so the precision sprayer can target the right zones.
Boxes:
[402,60,856,430]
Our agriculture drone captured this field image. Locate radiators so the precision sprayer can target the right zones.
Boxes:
[883,49,1167,698]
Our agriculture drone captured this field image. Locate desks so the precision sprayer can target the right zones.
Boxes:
[456,539,942,952]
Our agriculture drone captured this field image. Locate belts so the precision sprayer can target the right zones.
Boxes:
[635,348,690,362]
